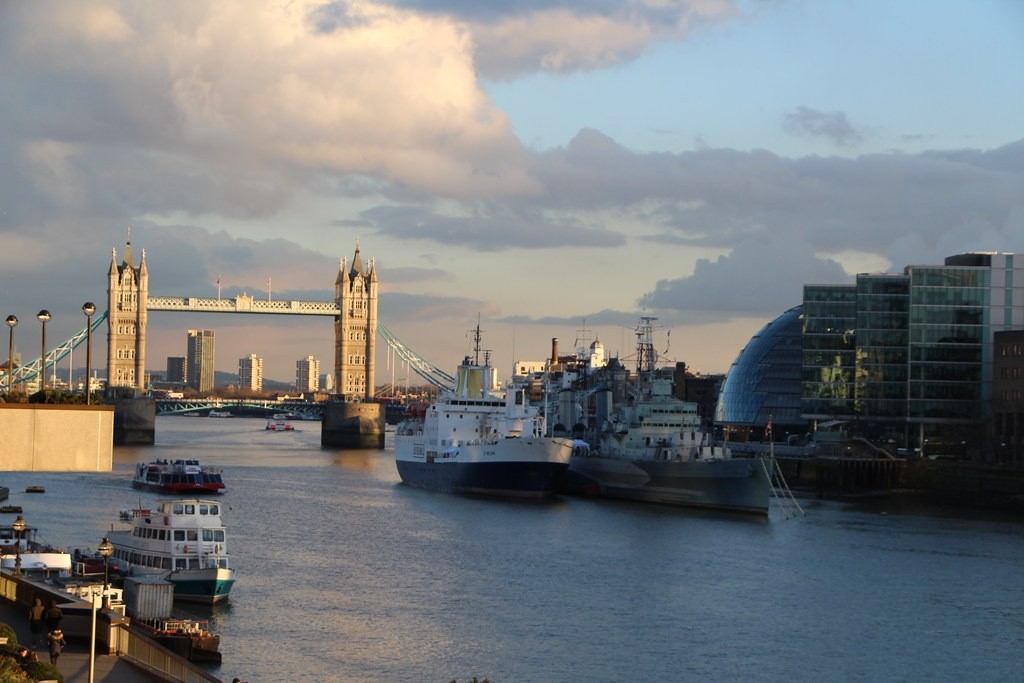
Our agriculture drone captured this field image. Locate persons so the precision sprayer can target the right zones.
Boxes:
[232,677,241,683]
[40,599,63,648]
[14,647,38,664]
[28,597,45,650]
[45,625,66,665]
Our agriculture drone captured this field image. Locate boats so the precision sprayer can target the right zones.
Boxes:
[184,409,236,418]
[395,354,573,501]
[68,499,236,605]
[132,457,229,495]
[573,364,775,518]
[263,418,296,431]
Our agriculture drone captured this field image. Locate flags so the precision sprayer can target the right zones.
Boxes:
[764,419,773,438]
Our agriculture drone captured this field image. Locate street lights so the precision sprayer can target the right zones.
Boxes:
[80,300,97,406]
[97,535,115,614]
[10,513,26,580]
[5,314,19,400]
[36,309,52,393]
